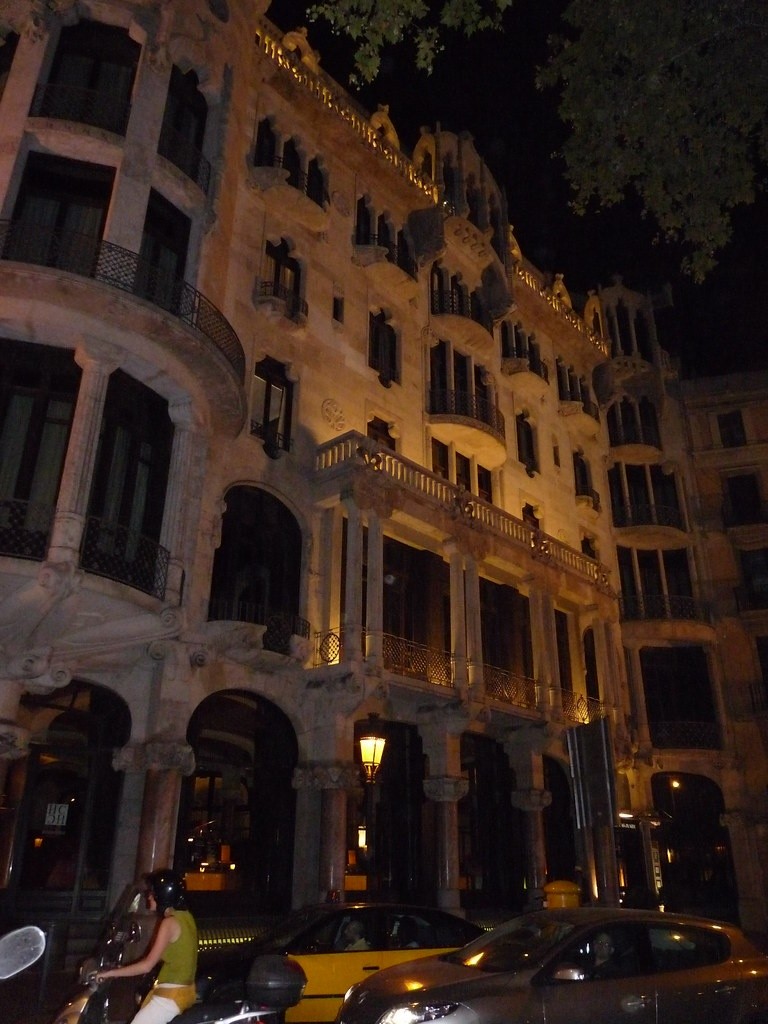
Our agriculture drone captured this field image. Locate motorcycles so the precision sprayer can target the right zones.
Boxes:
[56,876,308,1024]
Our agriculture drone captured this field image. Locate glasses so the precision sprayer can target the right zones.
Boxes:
[595,940,611,950]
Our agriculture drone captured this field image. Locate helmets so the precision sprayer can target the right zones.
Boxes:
[146,869,186,916]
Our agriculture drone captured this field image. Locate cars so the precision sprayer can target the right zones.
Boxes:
[225,899,501,1024]
[331,904,767,1024]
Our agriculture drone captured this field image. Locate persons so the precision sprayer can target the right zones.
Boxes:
[95,870,199,1024]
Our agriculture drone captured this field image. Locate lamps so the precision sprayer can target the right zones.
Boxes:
[358,736,386,786]
